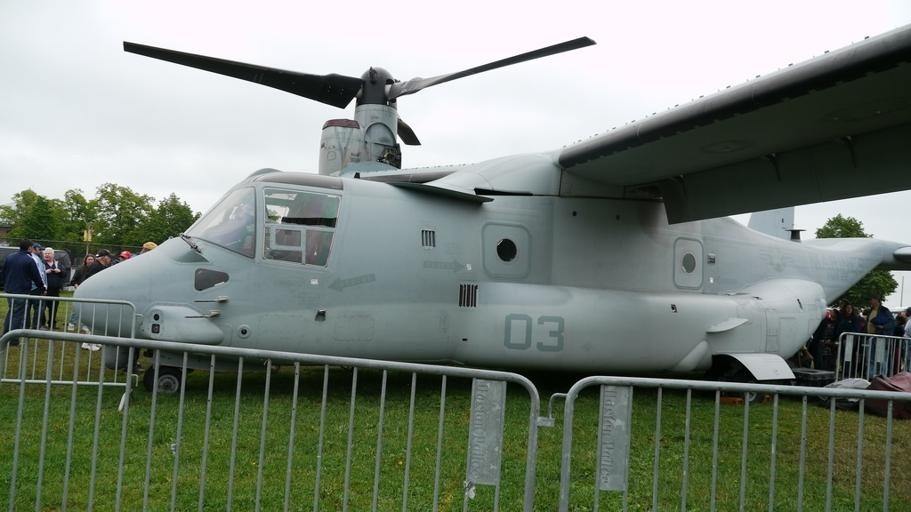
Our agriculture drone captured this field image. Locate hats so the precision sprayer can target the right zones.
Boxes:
[96,249,115,257]
[118,251,133,259]
[32,242,45,250]
[20,240,32,247]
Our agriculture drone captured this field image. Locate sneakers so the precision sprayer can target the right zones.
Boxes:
[81,343,102,351]
[68,323,91,334]
[33,325,56,331]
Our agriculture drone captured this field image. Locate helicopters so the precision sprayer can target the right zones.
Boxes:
[67,22,909,399]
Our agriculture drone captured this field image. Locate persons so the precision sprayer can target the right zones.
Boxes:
[38,246,68,329]
[81,248,113,351]
[67,254,95,331]
[19,243,46,326]
[138,242,159,255]
[117,250,132,262]
[1,237,48,347]
[802,292,911,382]
